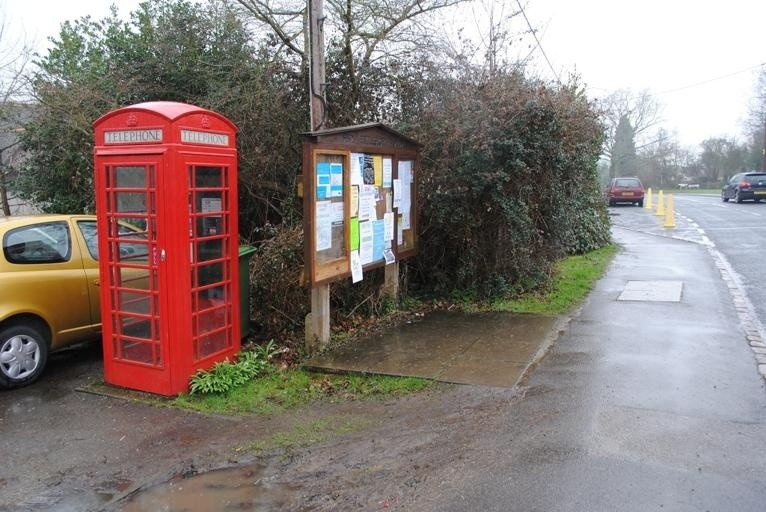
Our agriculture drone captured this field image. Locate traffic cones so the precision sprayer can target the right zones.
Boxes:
[655,190,666,216]
[664,194,676,227]
[645,187,653,210]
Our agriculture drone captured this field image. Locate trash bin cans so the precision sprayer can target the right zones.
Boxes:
[198,244,257,339]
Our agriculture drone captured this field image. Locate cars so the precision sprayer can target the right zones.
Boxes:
[603,176,645,208]
[677,181,700,189]
[0,214,193,389]
[722,172,766,203]
